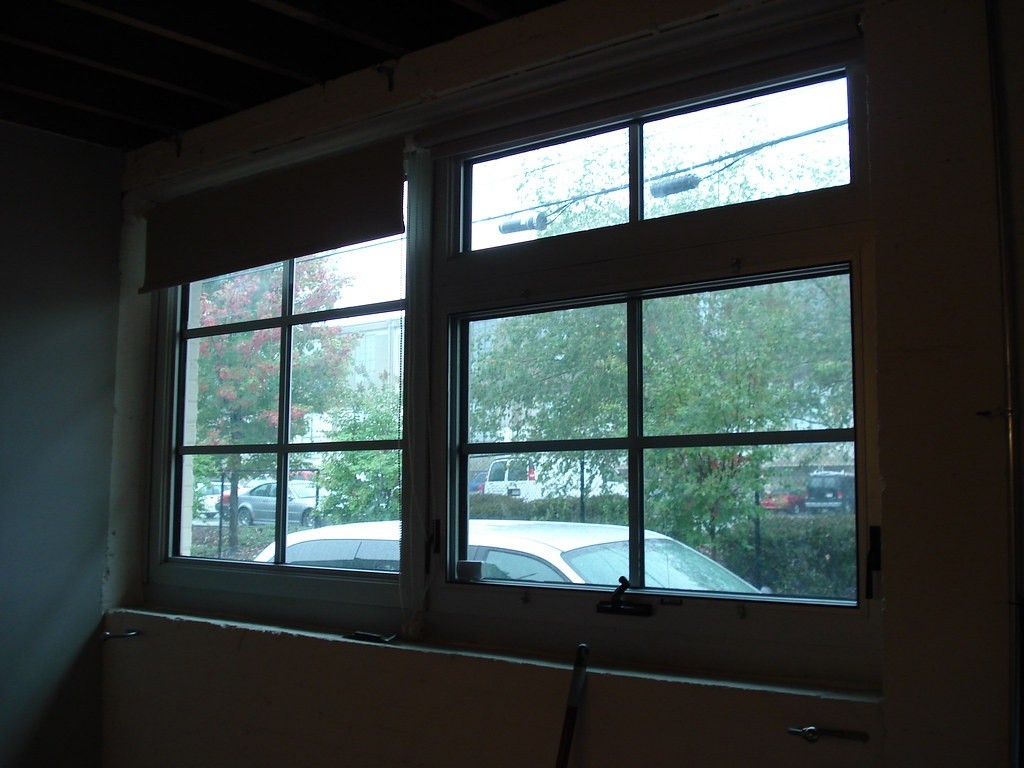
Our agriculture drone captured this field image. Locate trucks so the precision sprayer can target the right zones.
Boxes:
[803,469,855,514]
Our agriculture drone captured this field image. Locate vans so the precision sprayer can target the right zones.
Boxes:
[483,455,628,502]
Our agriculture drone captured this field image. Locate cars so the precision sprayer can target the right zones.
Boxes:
[468,470,487,495]
[251,517,775,596]
[760,487,807,513]
[194,470,352,528]
